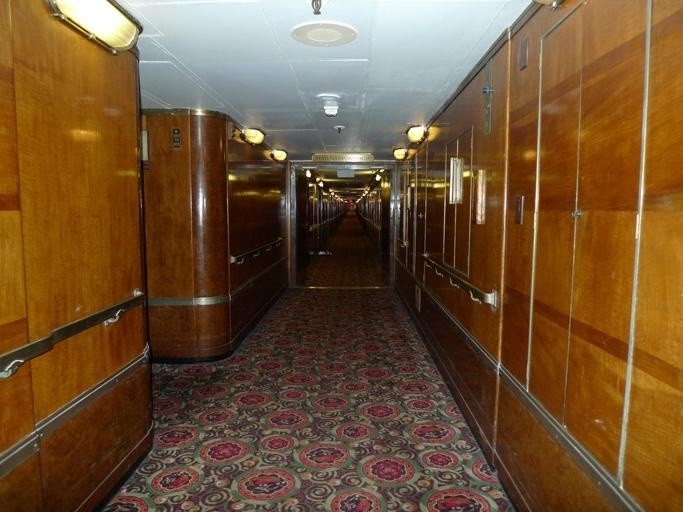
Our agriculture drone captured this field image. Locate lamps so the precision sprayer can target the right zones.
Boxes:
[49,0,144,55]
